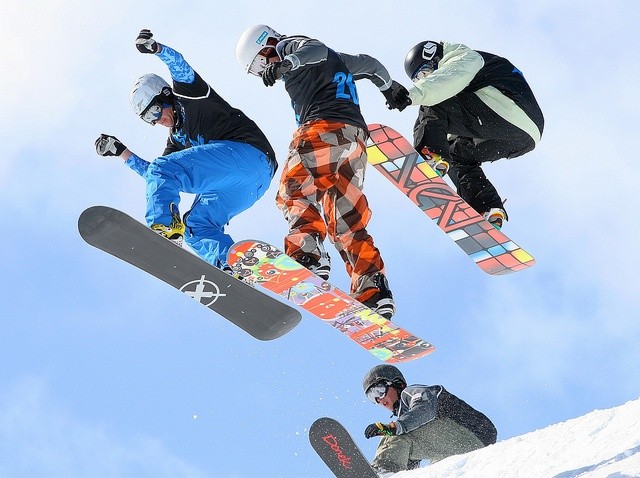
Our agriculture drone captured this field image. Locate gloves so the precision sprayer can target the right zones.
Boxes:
[135,29,158,54]
[365,422,396,439]
[94,134,128,156]
[262,59,293,87]
[380,80,413,112]
[385,96,412,112]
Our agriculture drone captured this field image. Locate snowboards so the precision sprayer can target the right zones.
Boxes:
[226,239,436,365]
[367,123,535,276]
[309,417,379,477]
[78,205,302,341]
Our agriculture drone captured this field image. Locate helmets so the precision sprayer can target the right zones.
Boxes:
[128,73,171,126]
[363,365,408,394]
[235,24,281,75]
[404,41,443,81]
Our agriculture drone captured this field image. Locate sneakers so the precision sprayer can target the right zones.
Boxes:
[152,216,185,245]
[421,145,450,178]
[297,236,331,280]
[481,208,506,231]
[349,274,395,320]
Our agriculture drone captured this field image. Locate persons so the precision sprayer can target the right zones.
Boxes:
[364,364,497,475]
[235,24,409,322]
[95,29,278,287]
[386,40,544,230]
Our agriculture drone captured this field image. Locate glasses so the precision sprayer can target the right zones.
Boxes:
[366,382,397,405]
[249,54,267,78]
